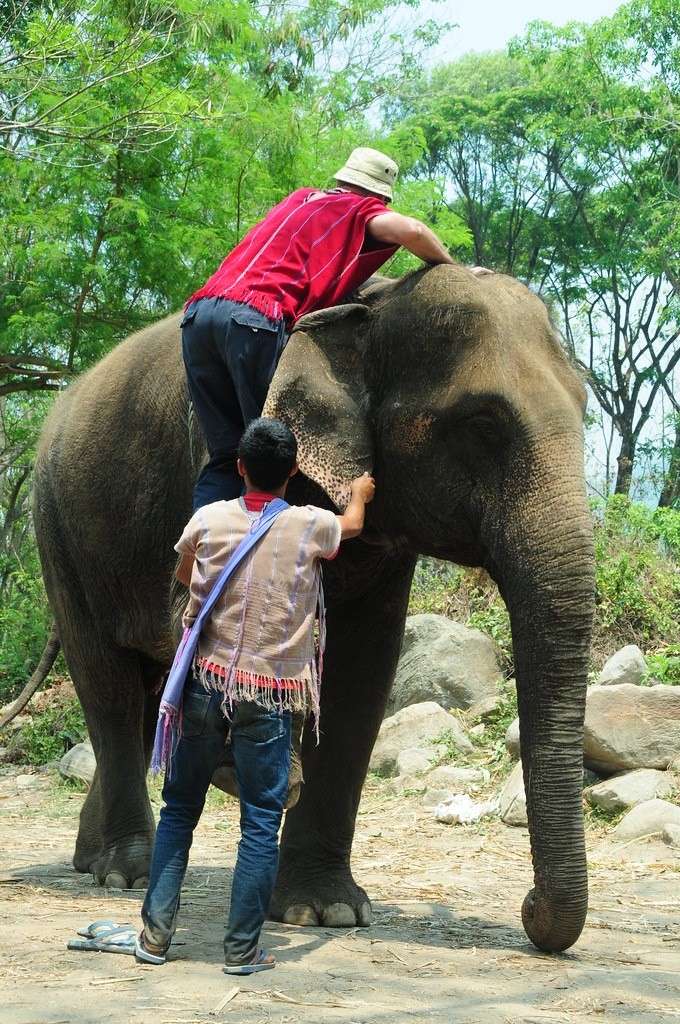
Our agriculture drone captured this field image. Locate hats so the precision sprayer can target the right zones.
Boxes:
[333,147,399,204]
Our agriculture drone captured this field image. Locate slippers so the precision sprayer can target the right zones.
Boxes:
[77,919,121,938]
[223,948,276,973]
[67,925,140,954]
[134,928,167,965]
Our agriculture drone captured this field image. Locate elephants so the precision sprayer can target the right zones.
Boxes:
[2,264,596,952]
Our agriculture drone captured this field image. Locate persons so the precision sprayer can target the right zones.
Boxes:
[180,145,495,513]
[134,417,376,972]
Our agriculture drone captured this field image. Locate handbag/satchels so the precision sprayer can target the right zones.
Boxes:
[150,627,200,779]
